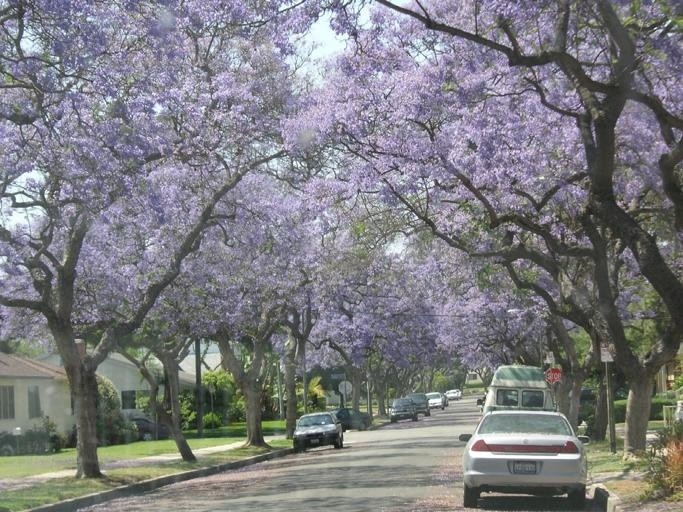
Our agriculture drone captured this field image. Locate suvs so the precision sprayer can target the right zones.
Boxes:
[405,393,431,417]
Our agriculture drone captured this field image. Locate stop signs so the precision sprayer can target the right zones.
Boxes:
[544,367,561,386]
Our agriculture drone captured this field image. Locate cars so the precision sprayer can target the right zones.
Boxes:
[330,407,373,433]
[388,397,419,423]
[292,411,343,454]
[425,388,462,411]
[459,409,590,508]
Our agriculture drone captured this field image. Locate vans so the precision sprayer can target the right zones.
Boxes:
[476,365,555,418]
